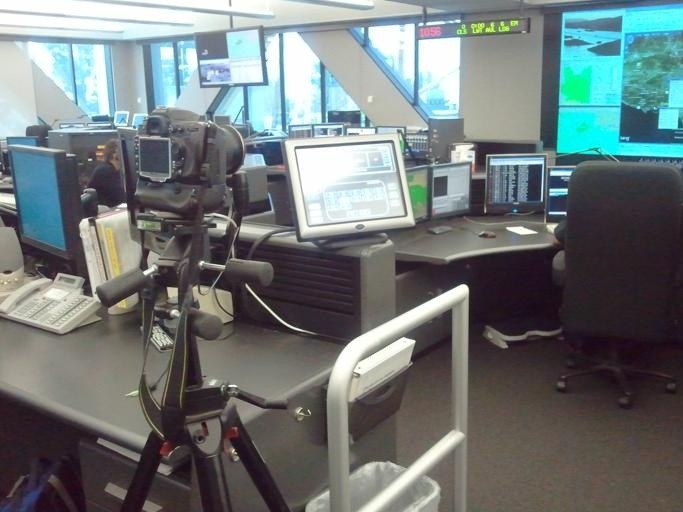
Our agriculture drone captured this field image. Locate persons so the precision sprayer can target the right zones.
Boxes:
[550,217,568,341]
[85,139,126,208]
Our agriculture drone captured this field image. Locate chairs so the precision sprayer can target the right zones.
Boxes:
[547,160,683,408]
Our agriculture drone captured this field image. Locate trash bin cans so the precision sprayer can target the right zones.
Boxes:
[305,461,442,512]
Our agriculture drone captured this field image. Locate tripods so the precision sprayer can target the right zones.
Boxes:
[98,225,293,511]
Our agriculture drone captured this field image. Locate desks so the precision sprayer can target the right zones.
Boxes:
[390,215,562,349]
[0,301,397,512]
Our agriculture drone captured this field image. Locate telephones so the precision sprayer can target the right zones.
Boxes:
[0,272,103,335]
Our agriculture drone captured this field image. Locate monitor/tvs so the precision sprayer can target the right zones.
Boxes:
[59,122,112,128]
[7,144,88,279]
[214,115,250,139]
[403,161,472,222]
[287,122,428,155]
[6,136,40,146]
[194,25,269,89]
[114,111,148,129]
[484,153,576,223]
[279,133,417,248]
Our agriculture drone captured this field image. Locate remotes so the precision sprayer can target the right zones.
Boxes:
[140,324,175,353]
